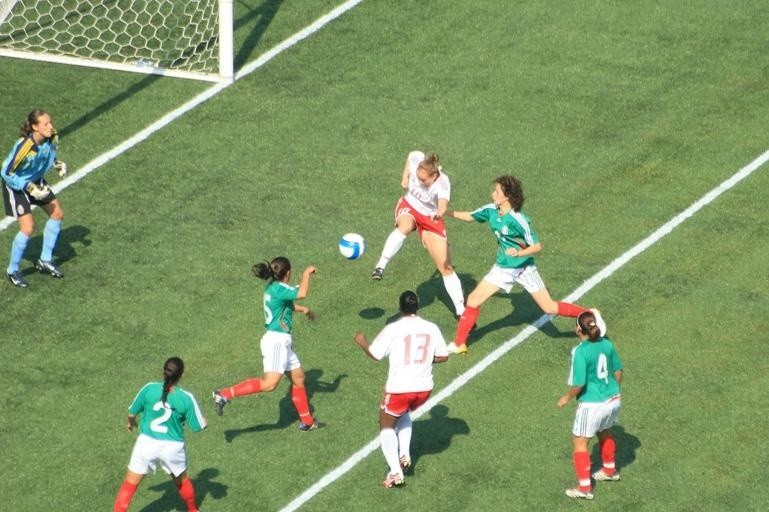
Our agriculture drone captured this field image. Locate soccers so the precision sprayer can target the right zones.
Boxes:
[339,233,365,258]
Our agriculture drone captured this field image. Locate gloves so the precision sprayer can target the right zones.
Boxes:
[53,160,67,177]
[24,181,50,200]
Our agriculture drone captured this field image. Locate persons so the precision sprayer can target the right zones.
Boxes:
[1,109,69,288]
[446,174,608,355]
[113,358,208,512]
[353,291,449,488]
[212,257,317,432]
[557,311,623,501]
[372,150,466,319]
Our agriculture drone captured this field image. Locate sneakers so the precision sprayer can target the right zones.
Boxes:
[399,454,411,473]
[565,485,594,499]
[591,468,620,481]
[381,472,405,488]
[371,267,384,281]
[212,389,228,416]
[298,418,328,433]
[589,308,606,338]
[5,270,29,288]
[446,343,468,355]
[34,258,65,278]
[455,314,477,334]
